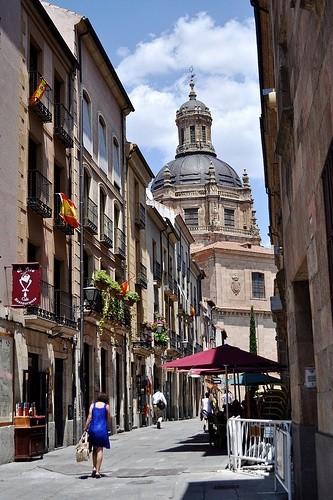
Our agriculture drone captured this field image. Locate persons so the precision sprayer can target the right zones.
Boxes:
[151,389,166,428]
[200,388,233,431]
[84,392,113,479]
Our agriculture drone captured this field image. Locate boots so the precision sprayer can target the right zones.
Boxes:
[202,424,205,430]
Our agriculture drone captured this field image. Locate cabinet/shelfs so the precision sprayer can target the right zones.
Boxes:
[14,416,46,461]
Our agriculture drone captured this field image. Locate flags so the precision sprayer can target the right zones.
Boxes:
[57,192,80,228]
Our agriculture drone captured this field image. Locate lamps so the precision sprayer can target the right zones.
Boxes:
[73,283,101,311]
[140,323,163,335]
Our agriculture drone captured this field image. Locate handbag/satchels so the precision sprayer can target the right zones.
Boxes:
[199,412,203,421]
[157,400,165,409]
[75,431,90,463]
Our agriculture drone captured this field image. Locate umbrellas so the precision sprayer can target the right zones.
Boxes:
[160,344,288,471]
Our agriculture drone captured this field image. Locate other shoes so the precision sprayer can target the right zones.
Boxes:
[92,468,101,478]
[157,422,160,428]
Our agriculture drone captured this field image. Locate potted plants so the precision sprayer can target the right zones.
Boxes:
[154,333,169,347]
[94,270,140,338]
